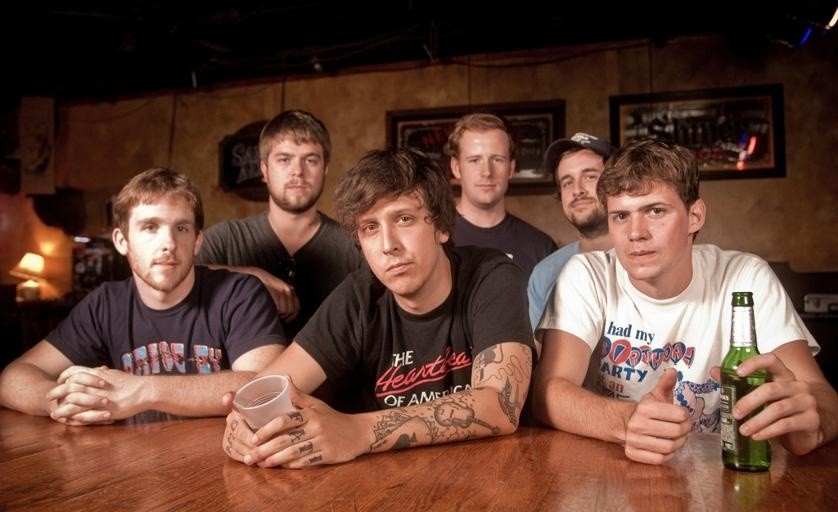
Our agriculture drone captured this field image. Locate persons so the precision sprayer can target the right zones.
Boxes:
[443,113,559,276]
[526,131,618,364]
[219,146,539,470]
[524,136,837,465]
[0,167,288,427]
[192,109,367,345]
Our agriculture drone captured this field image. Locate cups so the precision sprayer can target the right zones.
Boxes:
[232,374,295,434]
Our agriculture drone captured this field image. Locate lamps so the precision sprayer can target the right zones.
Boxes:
[8,253,45,303]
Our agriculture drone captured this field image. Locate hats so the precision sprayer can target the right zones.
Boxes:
[544,131,611,164]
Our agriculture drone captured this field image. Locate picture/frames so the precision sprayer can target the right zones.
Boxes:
[380,100,566,196]
[218,118,268,203]
[608,82,787,181]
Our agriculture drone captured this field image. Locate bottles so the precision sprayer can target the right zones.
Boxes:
[719,292,772,473]
